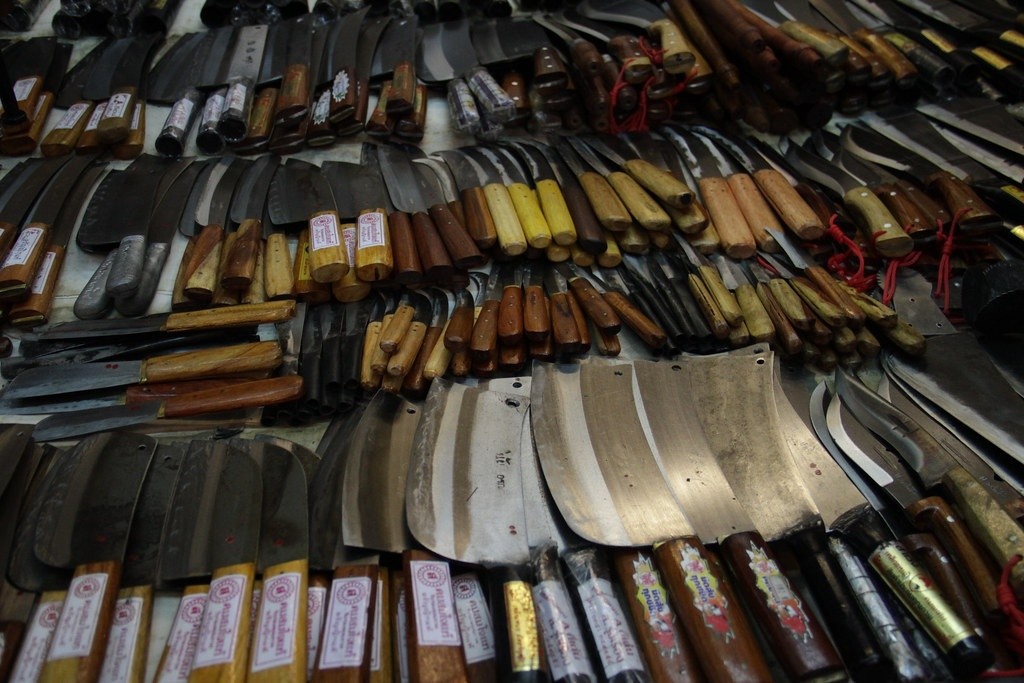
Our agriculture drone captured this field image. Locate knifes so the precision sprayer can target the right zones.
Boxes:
[0,0,1024,683]
